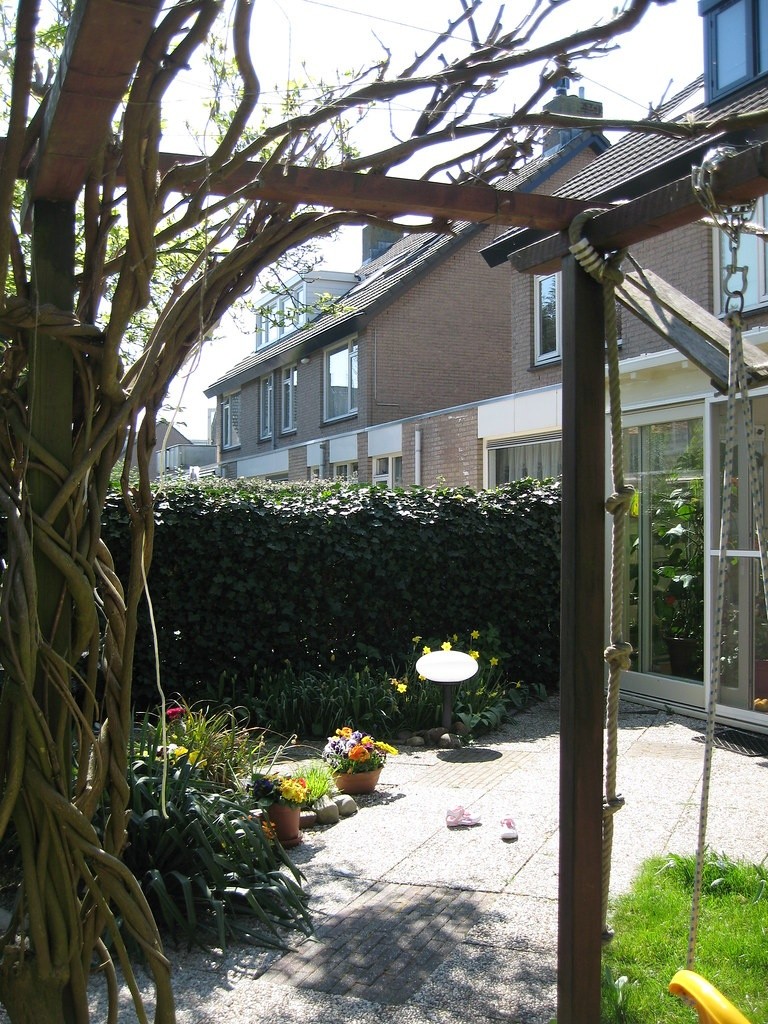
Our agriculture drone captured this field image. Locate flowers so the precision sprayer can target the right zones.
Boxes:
[321,727,398,773]
[245,772,312,810]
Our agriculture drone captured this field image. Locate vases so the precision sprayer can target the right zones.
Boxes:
[264,803,302,849]
[328,764,384,796]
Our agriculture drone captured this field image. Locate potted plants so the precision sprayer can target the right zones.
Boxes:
[634,439,768,713]
